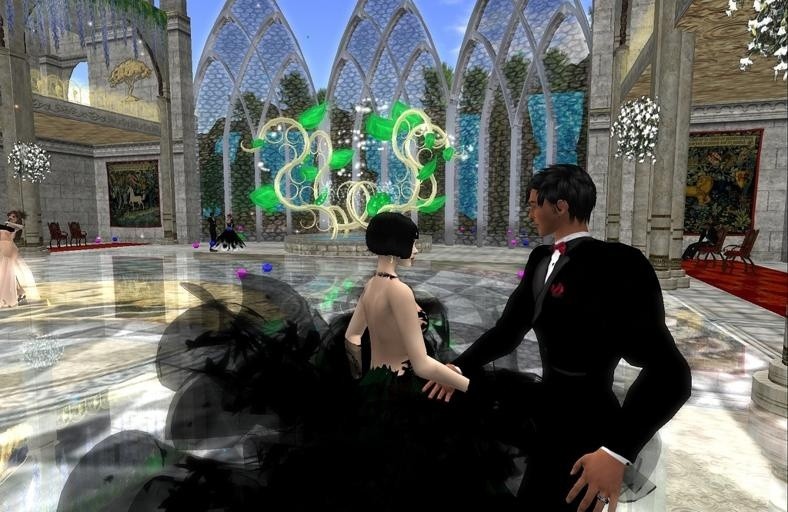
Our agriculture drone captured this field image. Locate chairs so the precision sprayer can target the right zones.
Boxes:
[697,228,729,260]
[724,230,760,266]
[47,222,68,248]
[68,222,87,247]
